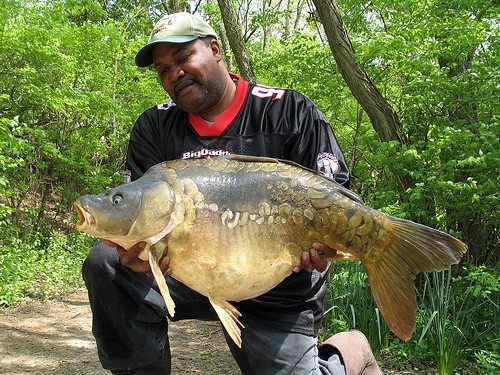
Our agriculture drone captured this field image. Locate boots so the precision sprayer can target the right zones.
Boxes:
[318,329,384,375]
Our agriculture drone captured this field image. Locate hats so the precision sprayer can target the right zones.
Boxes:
[134,12,219,68]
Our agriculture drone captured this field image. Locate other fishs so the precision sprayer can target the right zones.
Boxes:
[73,154,469,349]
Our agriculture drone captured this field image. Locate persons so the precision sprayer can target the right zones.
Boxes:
[81,12,385,375]
